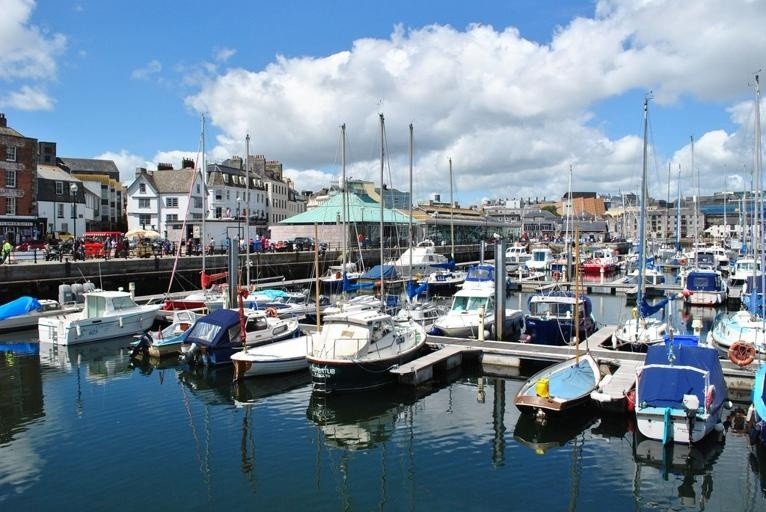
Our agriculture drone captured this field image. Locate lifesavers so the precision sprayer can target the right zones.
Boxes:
[729,341,756,366]
[267,307,277,317]
[628,390,635,411]
[706,384,716,413]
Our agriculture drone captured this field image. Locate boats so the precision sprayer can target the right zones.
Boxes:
[1,297,85,328]
[629,430,728,509]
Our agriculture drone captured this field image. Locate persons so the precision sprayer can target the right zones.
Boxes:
[311,238,315,251]
[43,234,144,262]
[152,238,175,256]
[1,240,11,264]
[181,237,216,256]
[224,234,305,255]
